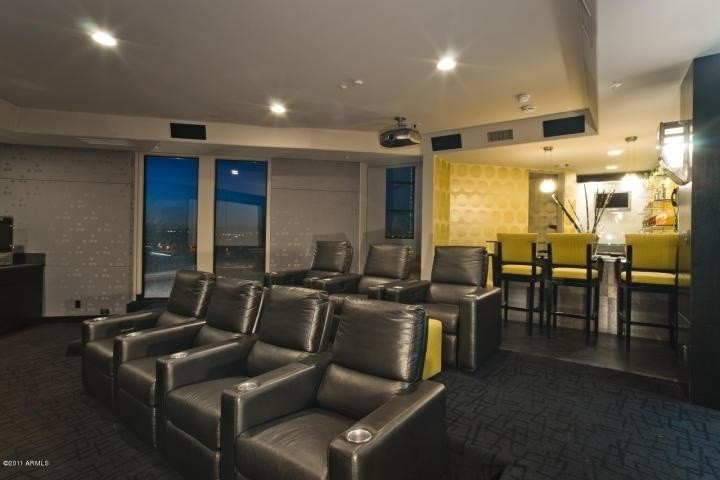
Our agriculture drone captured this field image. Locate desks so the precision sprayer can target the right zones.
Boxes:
[1,253,46,334]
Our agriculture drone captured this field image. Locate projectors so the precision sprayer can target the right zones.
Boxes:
[380,128,421,148]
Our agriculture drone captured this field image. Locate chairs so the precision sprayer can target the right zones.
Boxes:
[494,232,679,353]
[82,269,447,479]
[263,241,502,373]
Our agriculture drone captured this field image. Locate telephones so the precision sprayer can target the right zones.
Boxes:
[671,187,679,207]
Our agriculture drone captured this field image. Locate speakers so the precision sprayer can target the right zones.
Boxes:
[0,215,14,252]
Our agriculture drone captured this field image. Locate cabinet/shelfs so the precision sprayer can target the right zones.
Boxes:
[642,199,676,231]
